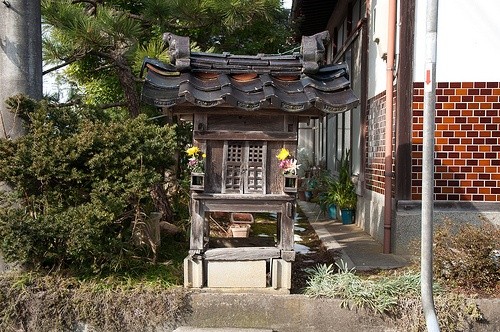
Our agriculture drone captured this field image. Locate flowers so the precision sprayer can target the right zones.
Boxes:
[275,147,302,188]
[184,146,206,185]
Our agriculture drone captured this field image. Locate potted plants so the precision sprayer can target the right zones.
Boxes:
[298,147,358,225]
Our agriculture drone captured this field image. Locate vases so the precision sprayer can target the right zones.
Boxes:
[230,224,251,237]
[231,212,254,224]
